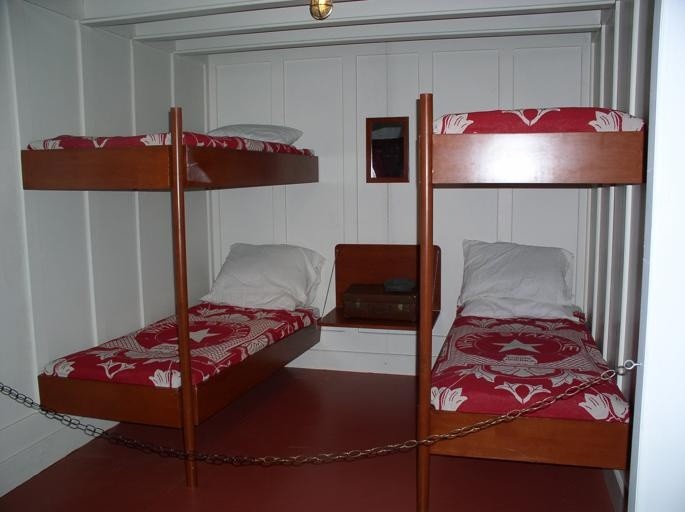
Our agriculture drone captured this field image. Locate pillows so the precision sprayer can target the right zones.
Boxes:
[205,124,302,145]
[196,242,325,313]
[457,238,579,323]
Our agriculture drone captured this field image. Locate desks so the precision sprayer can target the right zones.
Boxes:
[317,307,439,332]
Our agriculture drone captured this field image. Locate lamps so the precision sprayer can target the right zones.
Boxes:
[309,1,333,20]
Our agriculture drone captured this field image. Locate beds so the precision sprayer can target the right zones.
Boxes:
[21,106,321,488]
[407,94,645,512]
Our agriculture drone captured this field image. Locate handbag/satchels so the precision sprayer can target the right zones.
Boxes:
[384,274,414,292]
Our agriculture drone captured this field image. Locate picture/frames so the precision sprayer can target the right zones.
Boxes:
[365,116,409,183]
